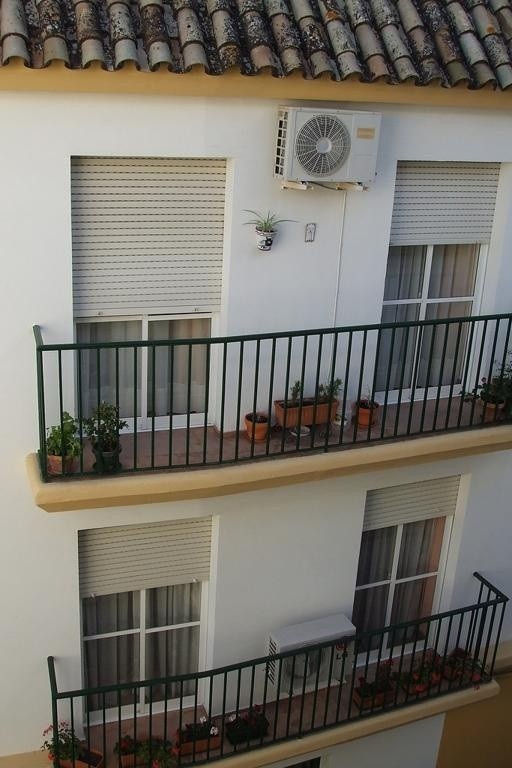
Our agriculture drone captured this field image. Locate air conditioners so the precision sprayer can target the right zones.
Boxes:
[263,612,359,699]
[272,107,382,194]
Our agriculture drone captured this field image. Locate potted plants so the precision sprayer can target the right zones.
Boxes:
[351,385,383,429]
[37,409,82,478]
[487,351,511,409]
[276,375,344,432]
[245,409,266,440]
[456,371,508,425]
[76,398,129,475]
[245,210,299,252]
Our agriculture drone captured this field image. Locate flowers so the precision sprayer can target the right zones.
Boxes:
[112,729,181,767]
[39,718,88,761]
[397,649,445,691]
[173,711,221,742]
[350,656,397,696]
[451,657,490,689]
[228,702,264,731]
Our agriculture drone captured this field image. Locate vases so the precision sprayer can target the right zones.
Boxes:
[223,718,270,745]
[118,751,150,767]
[351,686,396,710]
[60,747,105,767]
[177,734,220,756]
[442,663,459,677]
[403,680,416,696]
